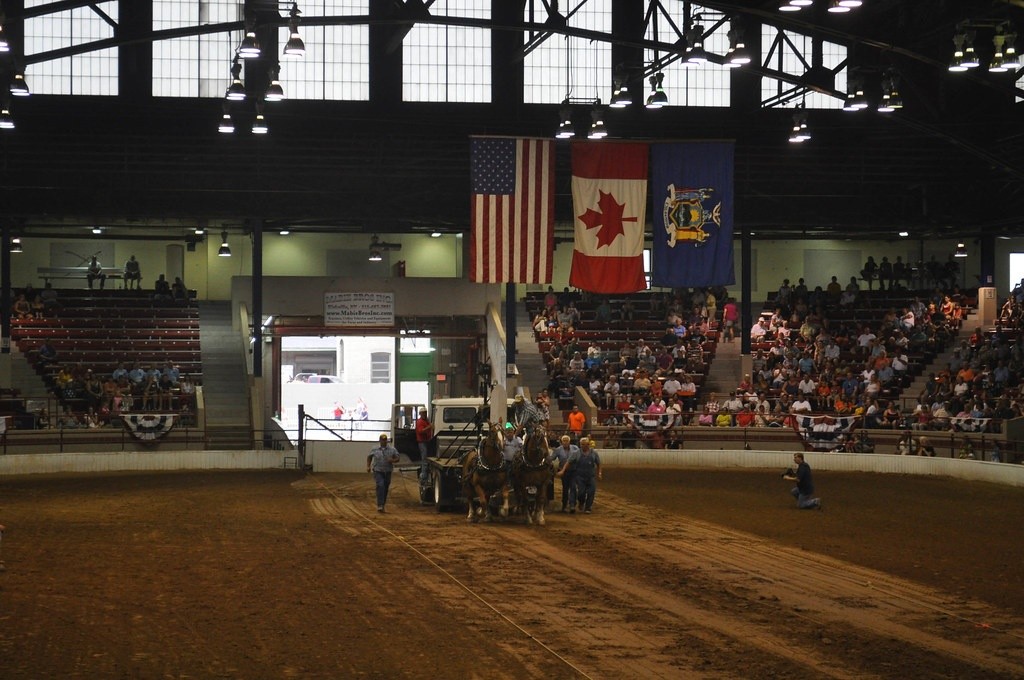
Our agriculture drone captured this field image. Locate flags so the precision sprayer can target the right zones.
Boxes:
[650,141,734,288]
[569,143,648,294]
[469,138,555,285]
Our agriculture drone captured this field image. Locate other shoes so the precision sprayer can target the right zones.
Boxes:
[562,507,567,512]
[584,505,591,514]
[815,498,821,510]
[571,507,575,513]
[377,505,385,513]
[579,503,584,510]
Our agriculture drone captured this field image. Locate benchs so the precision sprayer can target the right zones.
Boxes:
[0,261,202,431]
[523,265,1024,461]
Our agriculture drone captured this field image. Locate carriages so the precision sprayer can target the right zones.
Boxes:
[419,405,554,526]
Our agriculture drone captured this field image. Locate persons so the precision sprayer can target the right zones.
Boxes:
[38,408,49,429]
[416,408,434,483]
[334,398,368,419]
[556,438,602,515]
[783,453,820,510]
[10,283,57,320]
[55,360,195,429]
[565,404,585,438]
[504,396,540,487]
[38,338,56,365]
[544,435,579,513]
[533,285,732,428]
[87,256,106,289]
[124,255,142,289]
[367,434,400,513]
[155,274,188,304]
[722,254,1024,463]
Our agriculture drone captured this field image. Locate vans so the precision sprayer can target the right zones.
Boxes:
[391,398,516,459]
[295,373,345,383]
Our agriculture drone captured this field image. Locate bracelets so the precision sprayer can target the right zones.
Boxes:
[599,473,601,474]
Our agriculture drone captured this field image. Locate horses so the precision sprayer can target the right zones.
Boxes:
[509,419,553,526]
[461,416,510,524]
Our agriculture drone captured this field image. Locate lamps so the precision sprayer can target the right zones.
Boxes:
[834,52,903,114]
[554,55,665,143]
[0,24,32,130]
[952,240,969,257]
[368,233,382,261]
[10,235,23,253]
[91,225,102,234]
[776,0,864,16]
[789,102,812,147]
[676,5,752,70]
[218,228,232,257]
[279,225,290,236]
[194,225,205,235]
[946,19,1024,108]
[217,1,303,135]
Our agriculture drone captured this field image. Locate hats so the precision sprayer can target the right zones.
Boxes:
[419,408,427,415]
[513,394,523,403]
[380,434,387,440]
